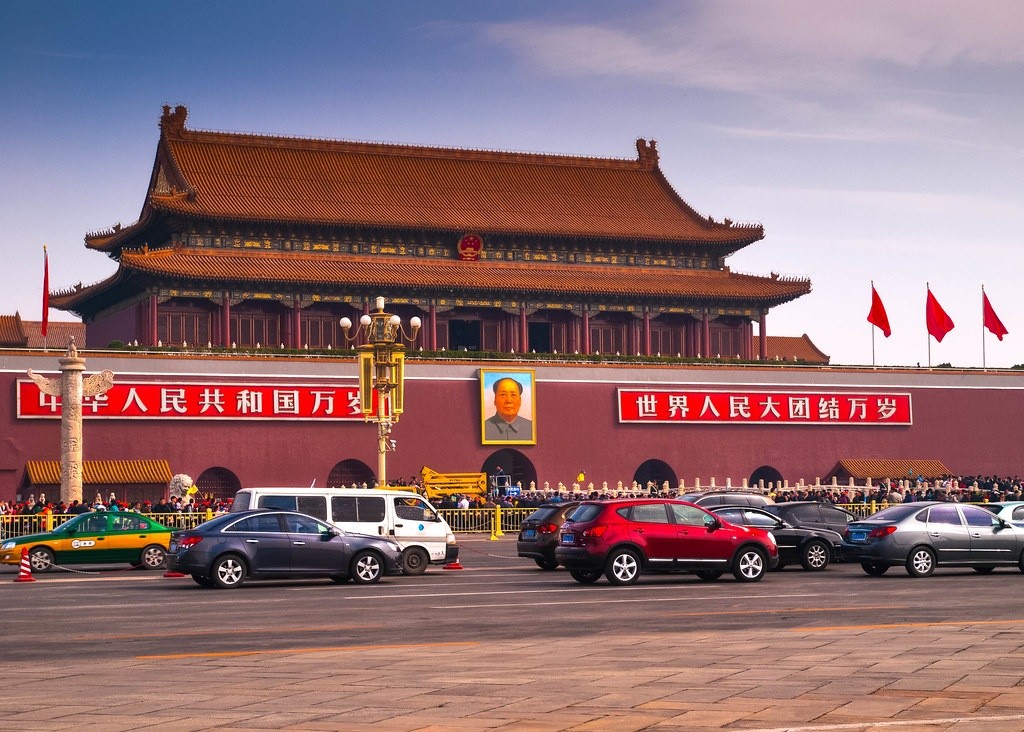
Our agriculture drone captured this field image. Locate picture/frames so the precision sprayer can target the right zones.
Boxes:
[481,368,537,444]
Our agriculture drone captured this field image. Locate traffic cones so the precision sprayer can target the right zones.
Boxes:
[163,570,185,577]
[13,547,36,582]
[443,554,464,570]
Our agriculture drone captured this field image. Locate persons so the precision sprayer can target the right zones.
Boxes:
[0,464,1024,541]
[484,372,532,440]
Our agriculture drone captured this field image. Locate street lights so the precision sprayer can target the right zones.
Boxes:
[340,297,422,490]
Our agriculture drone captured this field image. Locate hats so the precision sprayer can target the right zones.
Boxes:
[460,494,466,498]
[25,501,31,504]
[474,497,481,501]
[70,503,74,507]
[62,504,68,509]
[18,504,23,508]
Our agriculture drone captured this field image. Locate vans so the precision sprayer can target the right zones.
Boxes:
[230,487,459,576]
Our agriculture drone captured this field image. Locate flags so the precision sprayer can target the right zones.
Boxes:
[187,484,199,494]
[926,288,955,343]
[983,290,1009,341]
[867,286,892,338]
[40,254,50,340]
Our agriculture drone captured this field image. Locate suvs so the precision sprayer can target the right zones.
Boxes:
[674,491,776,511]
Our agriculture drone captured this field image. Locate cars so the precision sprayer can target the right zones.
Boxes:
[840,500,1024,578]
[554,498,780,586]
[165,507,403,590]
[760,500,862,540]
[0,505,184,574]
[517,501,582,570]
[975,501,1024,524]
[706,505,843,572]
